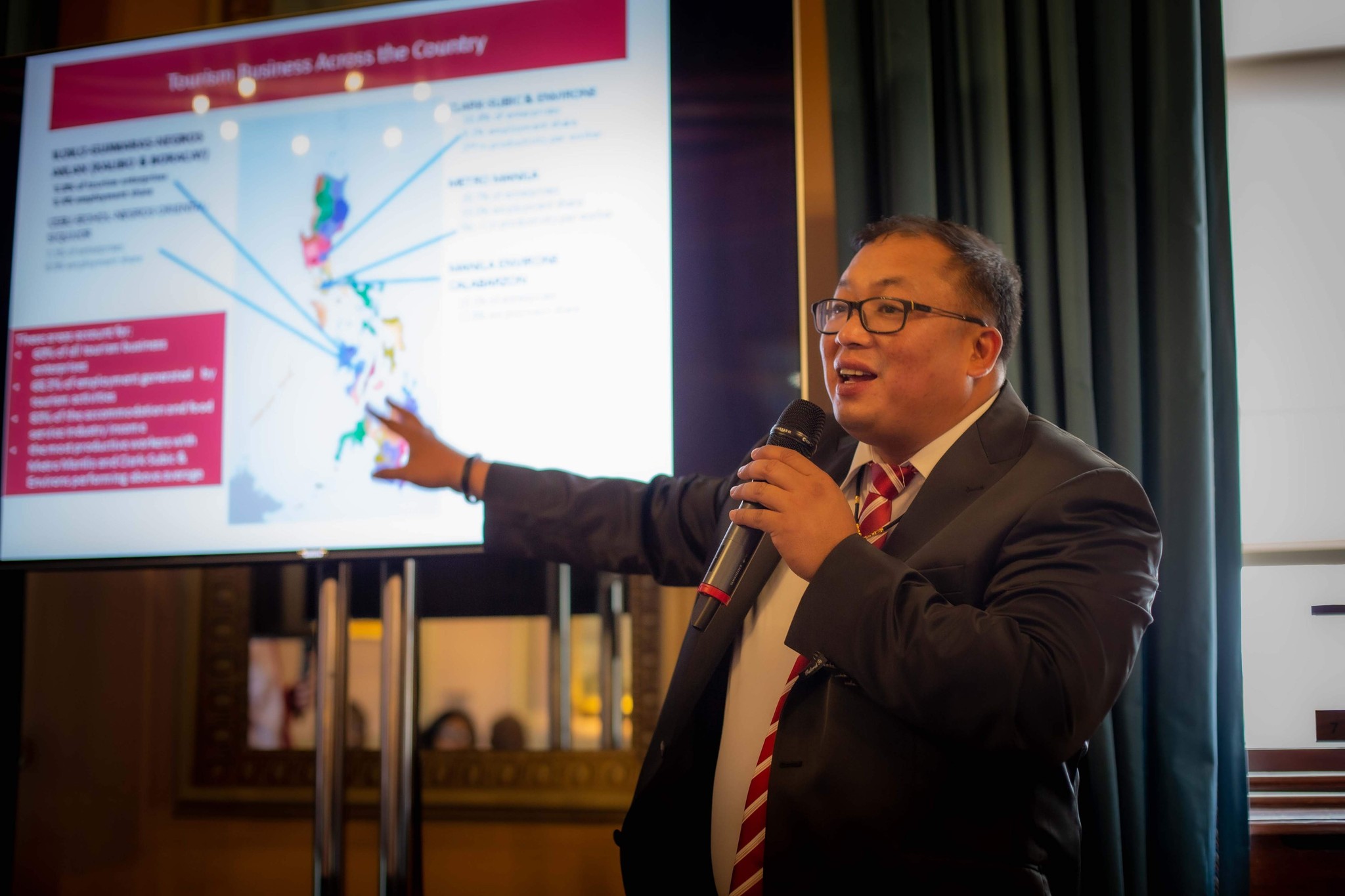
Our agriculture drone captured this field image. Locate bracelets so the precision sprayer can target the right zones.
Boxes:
[461,455,481,504]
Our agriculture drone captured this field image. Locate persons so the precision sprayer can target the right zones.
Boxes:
[489,716,525,750]
[424,711,477,751]
[365,217,1163,895]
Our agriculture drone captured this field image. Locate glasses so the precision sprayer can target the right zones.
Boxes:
[810,296,988,334]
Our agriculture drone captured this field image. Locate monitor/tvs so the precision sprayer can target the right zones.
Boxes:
[0,0,797,573]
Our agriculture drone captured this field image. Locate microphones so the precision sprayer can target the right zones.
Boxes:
[691,398,826,633]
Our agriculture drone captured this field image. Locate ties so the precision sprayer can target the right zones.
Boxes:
[728,465,918,896]
